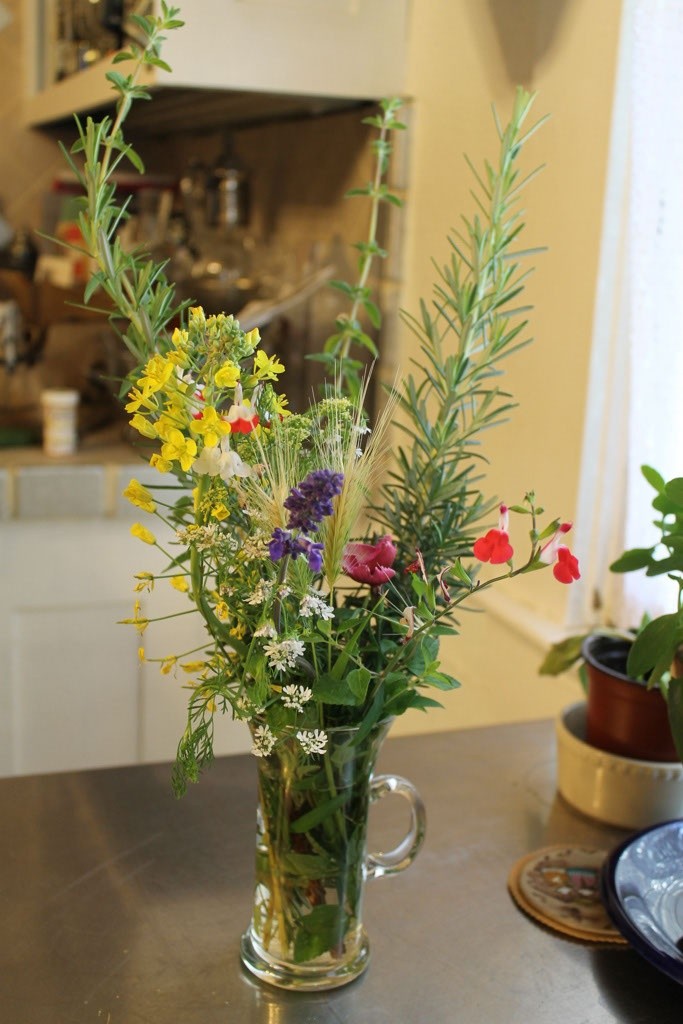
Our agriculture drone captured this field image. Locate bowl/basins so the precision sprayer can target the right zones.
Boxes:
[601,819,683,1005]
[555,701,683,831]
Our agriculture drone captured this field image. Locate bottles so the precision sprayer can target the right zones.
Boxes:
[42,390,80,455]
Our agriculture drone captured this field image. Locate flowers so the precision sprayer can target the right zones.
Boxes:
[44,0,580,785]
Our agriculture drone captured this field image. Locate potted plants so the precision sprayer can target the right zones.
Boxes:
[578,465,683,764]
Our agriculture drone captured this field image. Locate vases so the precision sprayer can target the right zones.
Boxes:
[239,715,429,990]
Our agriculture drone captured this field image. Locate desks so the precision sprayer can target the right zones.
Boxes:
[2,712,683,1023]
